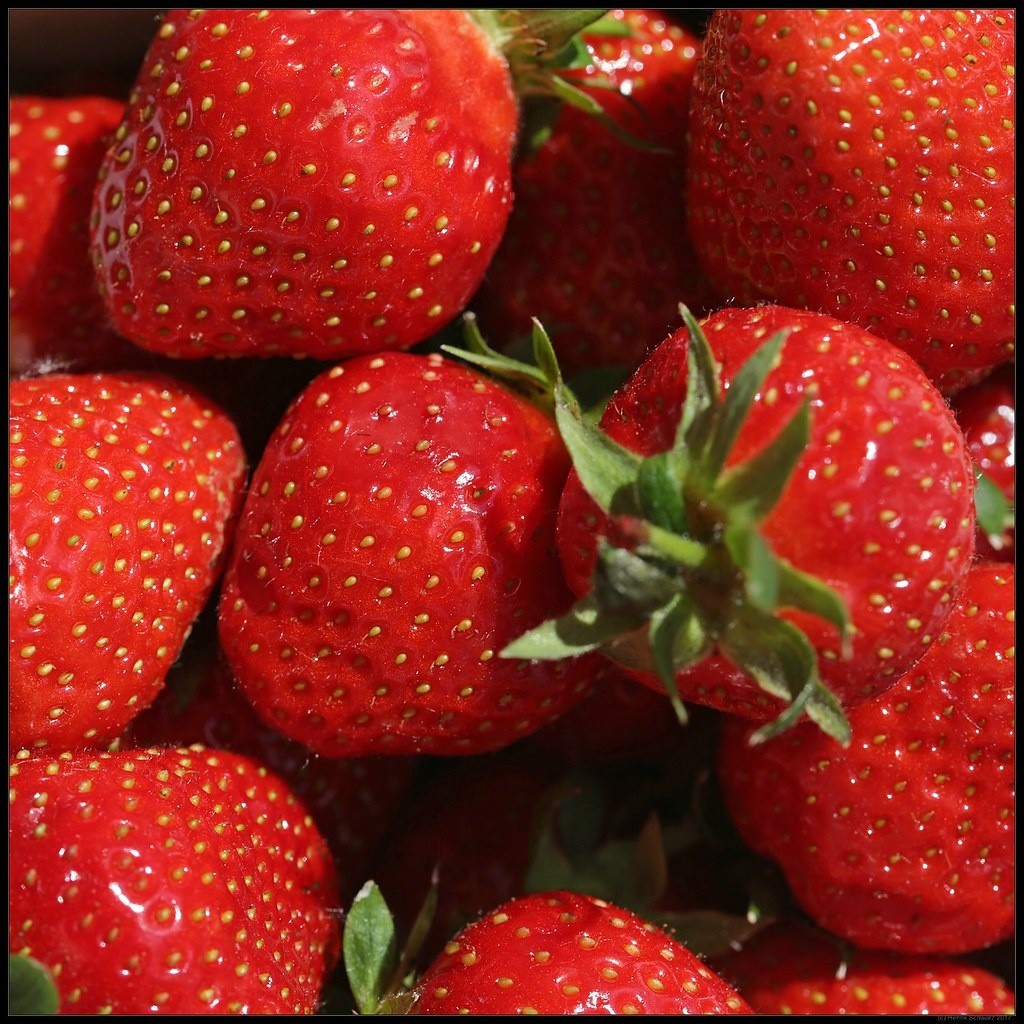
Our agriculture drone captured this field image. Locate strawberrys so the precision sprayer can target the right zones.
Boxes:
[10,8,1016,1015]
